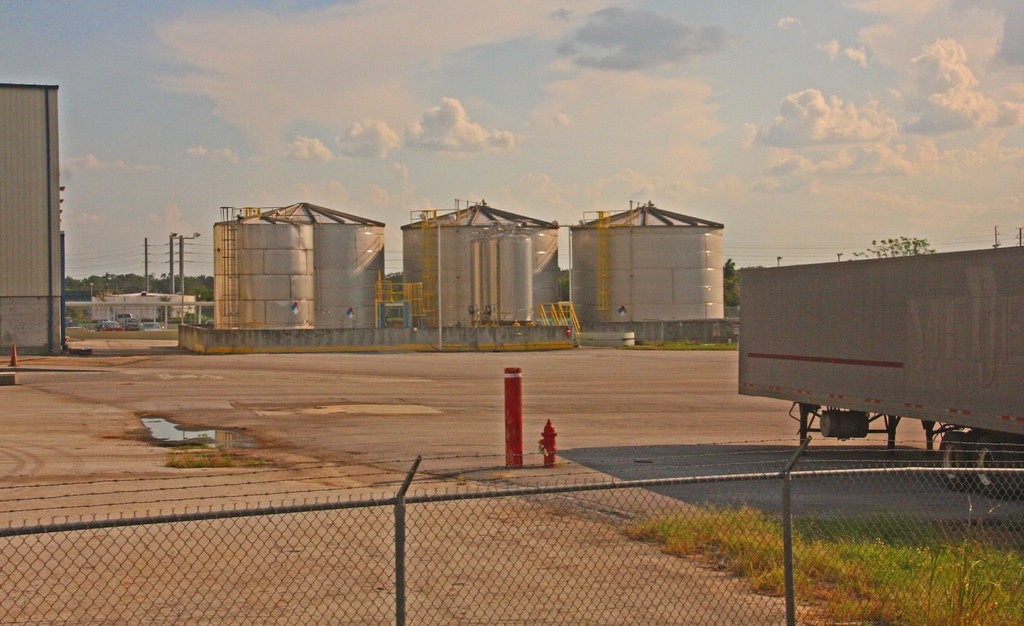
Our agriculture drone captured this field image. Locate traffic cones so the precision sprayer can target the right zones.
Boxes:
[8,343,19,367]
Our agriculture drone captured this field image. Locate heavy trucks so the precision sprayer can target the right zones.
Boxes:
[739,245,1023,493]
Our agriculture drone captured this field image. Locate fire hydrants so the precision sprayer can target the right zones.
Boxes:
[537,418,559,469]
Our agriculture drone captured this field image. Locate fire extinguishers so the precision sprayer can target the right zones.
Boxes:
[566,326,572,337]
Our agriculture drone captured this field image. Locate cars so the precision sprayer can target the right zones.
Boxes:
[64,316,73,327]
[204,320,214,327]
[139,322,165,333]
[97,312,141,333]
